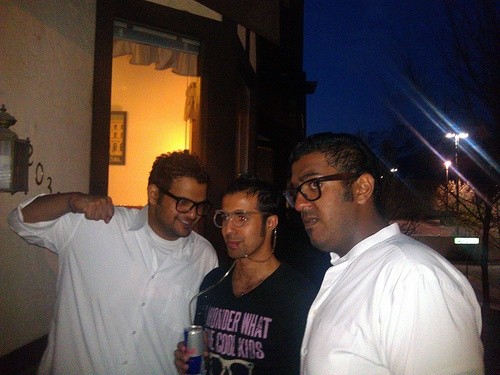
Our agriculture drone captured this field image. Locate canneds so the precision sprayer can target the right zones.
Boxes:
[183,325,209,375]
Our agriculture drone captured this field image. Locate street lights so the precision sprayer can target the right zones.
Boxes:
[444,131,469,235]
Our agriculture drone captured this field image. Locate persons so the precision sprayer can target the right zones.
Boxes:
[283,131,484,375]
[8,149,220,375]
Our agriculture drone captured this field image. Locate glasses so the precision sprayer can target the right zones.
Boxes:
[282,172,359,207]
[214,209,269,228]
[158,187,213,217]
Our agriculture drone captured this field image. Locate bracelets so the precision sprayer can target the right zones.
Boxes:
[173,173,320,375]
[67,192,81,213]
